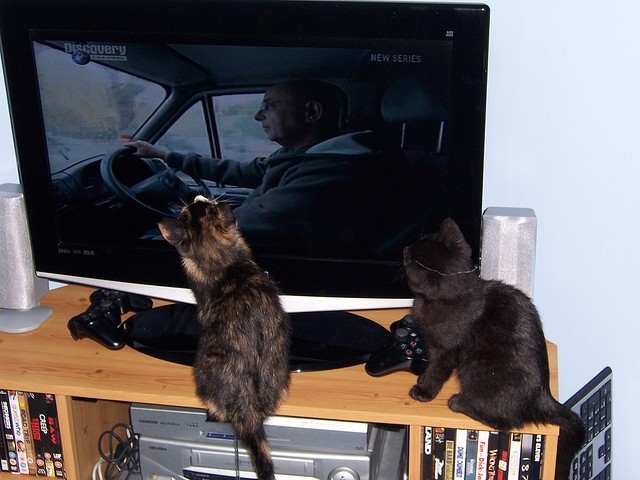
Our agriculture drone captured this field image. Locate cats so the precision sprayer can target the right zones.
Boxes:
[157,194,292,480]
[403,217,588,480]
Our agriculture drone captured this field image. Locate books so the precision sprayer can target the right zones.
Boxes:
[417,425,547,480]
[1,386,67,480]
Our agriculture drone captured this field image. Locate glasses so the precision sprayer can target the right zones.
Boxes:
[260,99,305,110]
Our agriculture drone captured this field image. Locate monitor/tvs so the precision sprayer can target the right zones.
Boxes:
[0,0,492,374]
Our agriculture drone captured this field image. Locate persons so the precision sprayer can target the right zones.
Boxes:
[126,61,425,271]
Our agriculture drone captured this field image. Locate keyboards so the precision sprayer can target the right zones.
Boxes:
[562,366,613,480]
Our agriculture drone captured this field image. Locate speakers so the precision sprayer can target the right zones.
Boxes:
[0,182,54,333]
[480,207,537,302]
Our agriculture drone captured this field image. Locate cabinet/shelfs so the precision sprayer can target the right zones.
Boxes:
[0,284,561,480]
